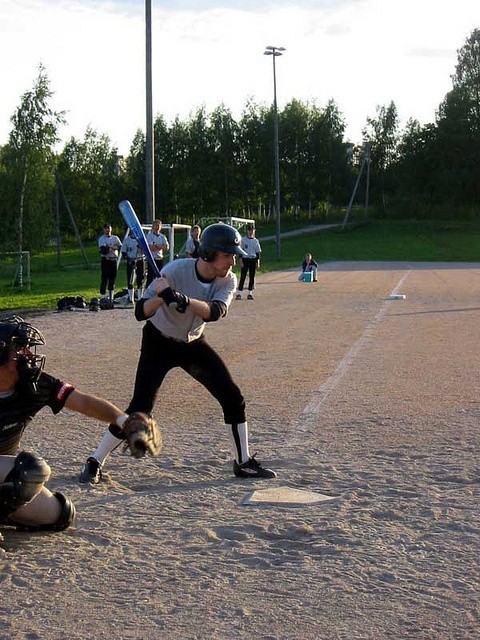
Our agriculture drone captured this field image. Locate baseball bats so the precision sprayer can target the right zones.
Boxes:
[118,200,178,308]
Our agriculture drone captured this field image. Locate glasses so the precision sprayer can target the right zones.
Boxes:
[104,225,109,228]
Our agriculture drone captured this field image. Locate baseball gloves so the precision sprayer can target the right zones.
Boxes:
[122,412,163,459]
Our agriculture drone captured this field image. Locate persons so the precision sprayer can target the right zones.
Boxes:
[236,224,262,301]
[186,225,201,257]
[79,223,276,482]
[147,220,169,288]
[99,224,122,302]
[121,230,144,303]
[298,253,317,282]
[0,317,151,532]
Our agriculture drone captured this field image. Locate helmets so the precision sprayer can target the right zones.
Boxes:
[197,223,249,261]
[0,317,44,397]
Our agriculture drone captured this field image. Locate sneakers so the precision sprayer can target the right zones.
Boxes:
[236,294,242,300]
[233,458,277,479]
[247,294,255,301]
[80,456,103,486]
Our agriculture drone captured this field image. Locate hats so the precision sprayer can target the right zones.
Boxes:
[103,223,111,228]
[248,225,257,231]
